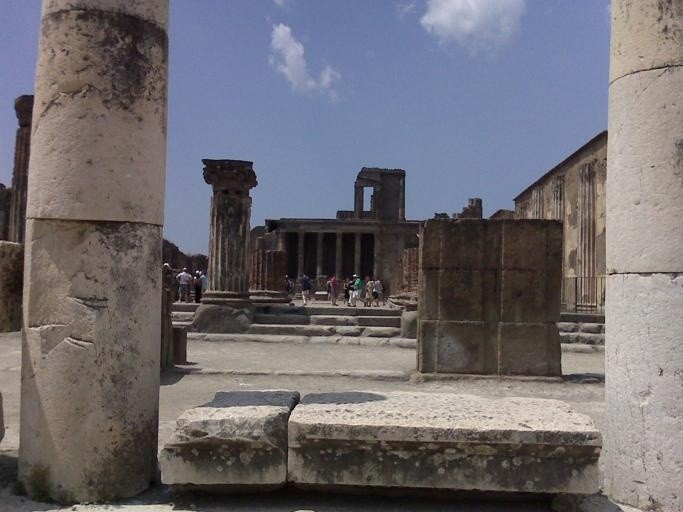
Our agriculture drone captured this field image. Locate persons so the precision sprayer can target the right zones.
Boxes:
[278,272,387,307]
[160,262,207,304]
